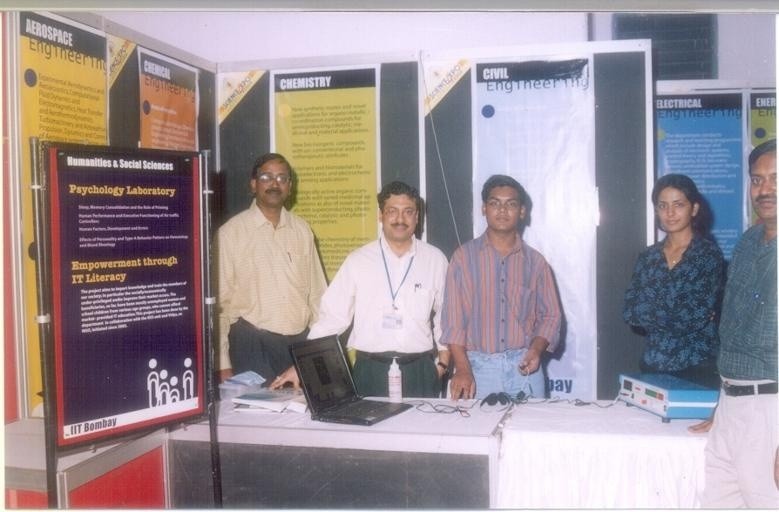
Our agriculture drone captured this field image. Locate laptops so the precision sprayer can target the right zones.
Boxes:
[288,335,413,425]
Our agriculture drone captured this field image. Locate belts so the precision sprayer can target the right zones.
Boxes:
[372,352,425,365]
[718,380,778,396]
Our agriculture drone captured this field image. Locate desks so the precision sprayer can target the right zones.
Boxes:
[163,391,713,509]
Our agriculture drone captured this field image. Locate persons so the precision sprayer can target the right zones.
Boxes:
[688,137,779,510]
[213,152,328,387]
[437,174,563,402]
[268,178,450,399]
[621,173,726,392]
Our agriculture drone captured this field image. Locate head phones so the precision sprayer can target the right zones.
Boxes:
[479,393,510,414]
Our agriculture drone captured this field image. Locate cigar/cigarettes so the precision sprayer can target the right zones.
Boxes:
[275,374,283,389]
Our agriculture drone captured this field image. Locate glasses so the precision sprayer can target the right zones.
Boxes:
[257,172,288,185]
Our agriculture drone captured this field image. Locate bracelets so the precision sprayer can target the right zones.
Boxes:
[436,361,446,369]
